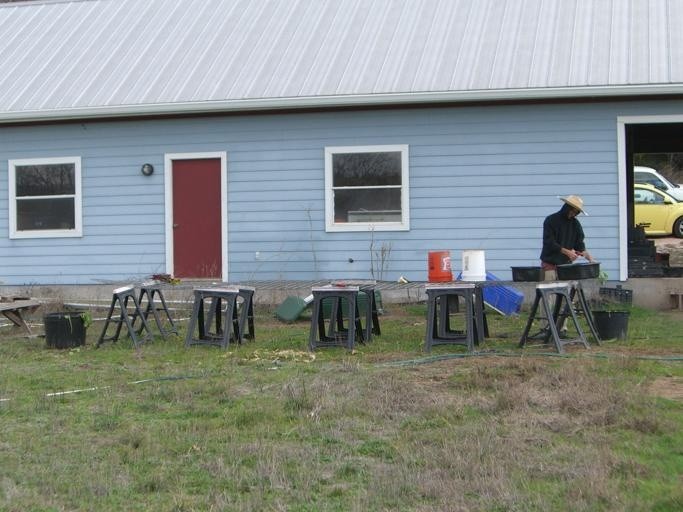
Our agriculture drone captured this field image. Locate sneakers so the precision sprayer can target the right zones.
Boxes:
[540,329,567,337]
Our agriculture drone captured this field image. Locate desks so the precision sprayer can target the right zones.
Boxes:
[0,298,42,335]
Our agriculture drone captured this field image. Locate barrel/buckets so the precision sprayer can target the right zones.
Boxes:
[428,251,452,283]
[461,250,487,281]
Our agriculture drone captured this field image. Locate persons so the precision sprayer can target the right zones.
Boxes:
[531,195,592,340]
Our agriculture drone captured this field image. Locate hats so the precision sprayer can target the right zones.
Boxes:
[558,195,588,216]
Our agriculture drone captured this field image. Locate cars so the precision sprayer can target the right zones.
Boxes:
[634,166,683,238]
